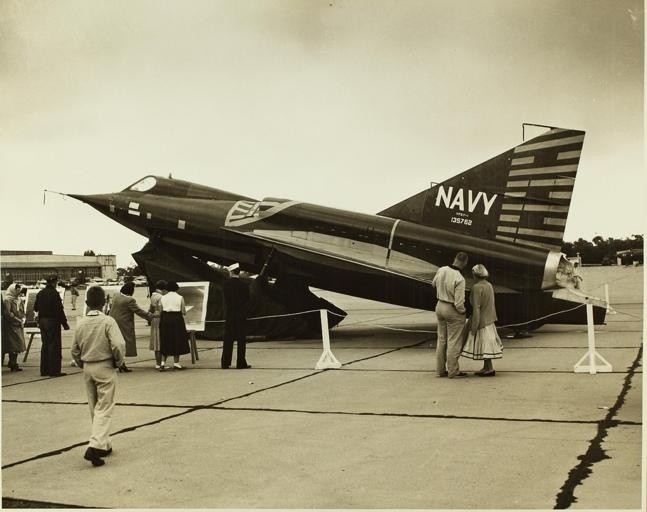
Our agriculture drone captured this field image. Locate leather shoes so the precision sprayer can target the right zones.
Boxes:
[237,364,252,369]
[155,362,186,370]
[84,447,113,467]
[8,362,22,372]
[436,371,449,377]
[222,365,236,369]
[41,372,50,376]
[454,373,468,379]
[474,368,496,377]
[50,373,67,377]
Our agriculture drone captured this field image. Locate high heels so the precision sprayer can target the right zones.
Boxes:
[119,363,133,374]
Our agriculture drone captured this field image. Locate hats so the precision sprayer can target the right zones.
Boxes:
[228,262,240,271]
[471,263,489,277]
[47,274,57,282]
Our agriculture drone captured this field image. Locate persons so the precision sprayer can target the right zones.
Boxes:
[460,264,504,376]
[146,279,169,372]
[572,261,583,290]
[1,293,10,367]
[71,284,128,469]
[33,274,71,377]
[55,283,65,304]
[109,280,153,373]
[430,250,469,378]
[221,260,252,370]
[69,284,79,311]
[157,280,191,372]
[3,282,27,373]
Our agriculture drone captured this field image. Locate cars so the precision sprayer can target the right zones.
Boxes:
[16,273,148,289]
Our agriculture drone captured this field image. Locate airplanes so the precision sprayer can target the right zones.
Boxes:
[38,121,606,341]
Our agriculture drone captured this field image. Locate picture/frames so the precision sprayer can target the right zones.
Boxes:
[23,287,66,332]
[83,285,123,317]
[176,281,210,331]
